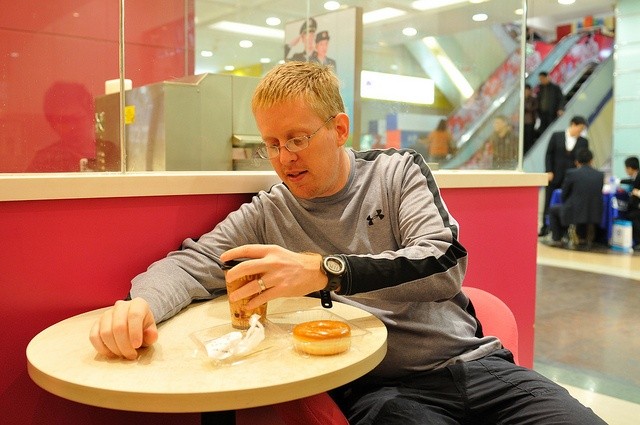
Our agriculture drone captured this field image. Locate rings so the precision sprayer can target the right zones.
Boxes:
[257,279,266,291]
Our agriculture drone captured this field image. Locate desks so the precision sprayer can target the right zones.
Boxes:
[24,293,388,425]
[545,186,630,239]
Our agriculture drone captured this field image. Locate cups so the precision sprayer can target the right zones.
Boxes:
[220,256,267,331]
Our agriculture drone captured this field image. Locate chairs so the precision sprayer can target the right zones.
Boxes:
[297,286,521,425]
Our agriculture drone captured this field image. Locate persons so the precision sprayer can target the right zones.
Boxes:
[620,157,639,251]
[547,148,605,247]
[538,115,590,238]
[311,31,336,76]
[535,71,564,126]
[491,114,518,170]
[428,118,453,162]
[90,59,610,424]
[284,17,317,62]
[524,84,537,152]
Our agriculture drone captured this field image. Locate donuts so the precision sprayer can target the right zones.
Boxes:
[293,320,354,356]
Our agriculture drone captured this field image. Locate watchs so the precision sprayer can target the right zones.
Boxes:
[316,250,346,309]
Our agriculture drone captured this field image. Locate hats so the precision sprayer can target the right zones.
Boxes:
[300,18,317,34]
[315,31,329,43]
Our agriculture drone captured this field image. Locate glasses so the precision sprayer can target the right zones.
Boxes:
[257,113,338,159]
